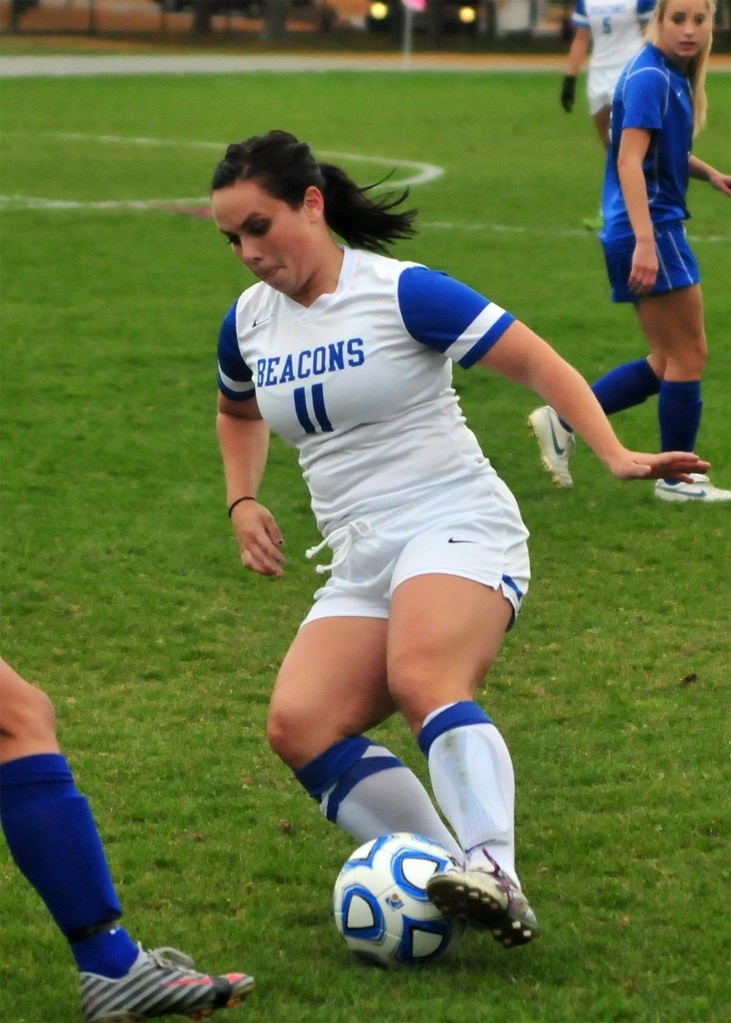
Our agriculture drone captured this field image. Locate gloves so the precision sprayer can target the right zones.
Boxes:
[560,75,577,112]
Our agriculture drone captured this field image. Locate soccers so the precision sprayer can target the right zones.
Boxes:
[332,831,466,970]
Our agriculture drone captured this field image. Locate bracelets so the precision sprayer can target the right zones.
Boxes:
[228,496,254,518]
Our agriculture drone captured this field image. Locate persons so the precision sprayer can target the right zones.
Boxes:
[0,659,253,1022]
[210,127,709,945]
[527,0,731,501]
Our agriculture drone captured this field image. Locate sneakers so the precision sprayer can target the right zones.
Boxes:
[655,472,731,501]
[425,847,540,949]
[79,942,256,1023]
[526,404,576,487]
